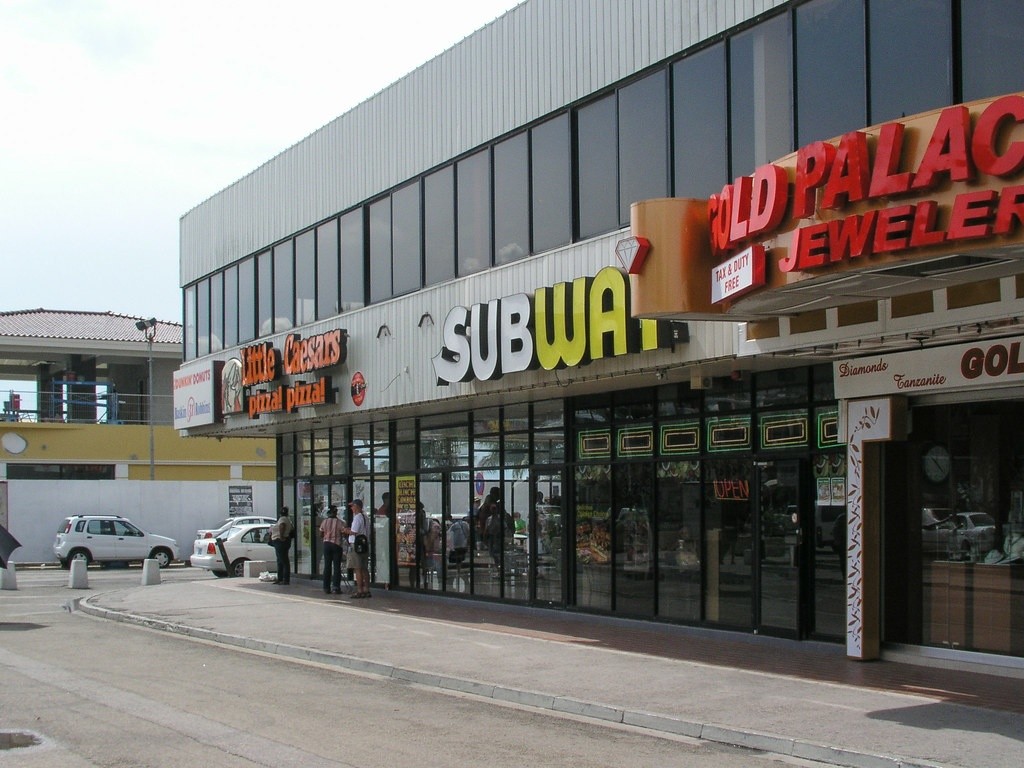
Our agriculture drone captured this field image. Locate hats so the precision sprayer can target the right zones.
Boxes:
[348,499,363,508]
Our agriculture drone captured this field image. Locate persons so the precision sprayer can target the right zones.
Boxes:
[379,492,389,515]
[271,506,292,585]
[447,519,468,585]
[424,519,443,584]
[319,508,346,594]
[419,487,550,578]
[484,506,511,579]
[342,498,372,599]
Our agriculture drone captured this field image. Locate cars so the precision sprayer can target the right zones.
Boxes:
[197,516,276,539]
[190,524,277,577]
[914,508,997,557]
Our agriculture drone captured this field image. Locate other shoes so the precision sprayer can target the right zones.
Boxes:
[351,591,365,598]
[491,572,500,578]
[364,592,372,598]
[332,589,344,594]
[323,588,331,594]
[278,580,289,585]
[273,580,281,584]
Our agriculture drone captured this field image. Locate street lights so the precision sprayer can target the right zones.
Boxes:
[135,317,157,481]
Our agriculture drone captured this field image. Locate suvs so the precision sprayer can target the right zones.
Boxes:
[779,504,848,551]
[54,514,178,568]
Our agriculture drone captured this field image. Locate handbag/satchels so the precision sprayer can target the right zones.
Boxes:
[355,533,369,553]
[289,527,295,538]
[449,548,466,563]
